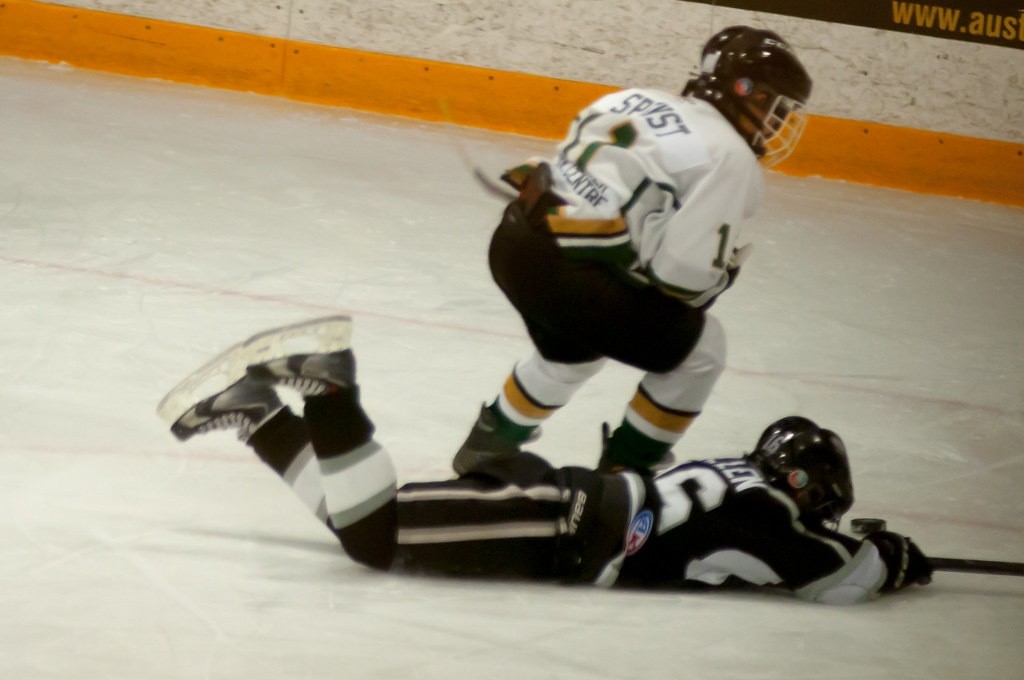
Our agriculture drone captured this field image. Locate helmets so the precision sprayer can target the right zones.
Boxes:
[745,416,853,524]
[694,25,812,169]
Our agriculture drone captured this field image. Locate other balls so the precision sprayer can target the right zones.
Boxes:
[849,517,887,535]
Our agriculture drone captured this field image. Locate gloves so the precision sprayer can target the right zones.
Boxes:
[863,531,931,594]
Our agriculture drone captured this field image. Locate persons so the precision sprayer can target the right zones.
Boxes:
[170,348,936,608]
[451,23,815,482]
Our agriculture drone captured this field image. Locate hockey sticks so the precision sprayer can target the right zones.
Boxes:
[926,552,1024,578]
[448,140,521,207]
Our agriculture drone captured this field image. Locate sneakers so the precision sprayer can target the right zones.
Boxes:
[156,342,282,441]
[453,401,541,475]
[243,315,356,395]
[599,423,673,479]
